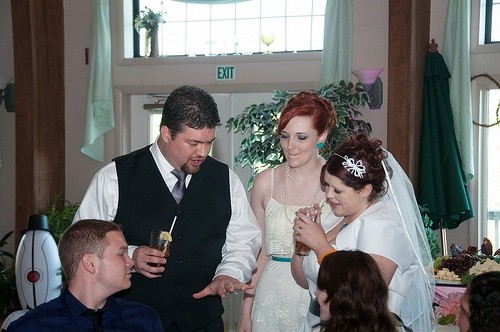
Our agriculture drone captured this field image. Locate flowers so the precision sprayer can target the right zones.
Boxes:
[134,2,167,40]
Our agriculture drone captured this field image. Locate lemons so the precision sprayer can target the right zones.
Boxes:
[160,231,172,241]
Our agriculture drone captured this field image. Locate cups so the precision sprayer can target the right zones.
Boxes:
[293,214,315,256]
[147,230,172,273]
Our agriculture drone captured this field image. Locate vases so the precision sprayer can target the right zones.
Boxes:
[149,32,160,57]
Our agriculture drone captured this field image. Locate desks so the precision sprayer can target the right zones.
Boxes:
[432,278,469,326]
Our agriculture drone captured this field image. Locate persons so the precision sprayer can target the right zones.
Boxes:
[291,132,438,332]
[236,91,345,332]
[6,219,166,332]
[70,84,264,332]
[314,249,413,332]
[455,271,500,332]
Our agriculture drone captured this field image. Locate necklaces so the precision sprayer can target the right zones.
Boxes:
[284,156,326,225]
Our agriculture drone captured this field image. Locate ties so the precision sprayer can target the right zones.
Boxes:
[168,168,188,205]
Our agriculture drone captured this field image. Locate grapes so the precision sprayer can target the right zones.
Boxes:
[434,254,481,275]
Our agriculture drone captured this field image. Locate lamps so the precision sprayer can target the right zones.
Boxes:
[351,67,384,110]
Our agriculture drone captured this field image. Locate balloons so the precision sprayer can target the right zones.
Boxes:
[261,32,275,46]
